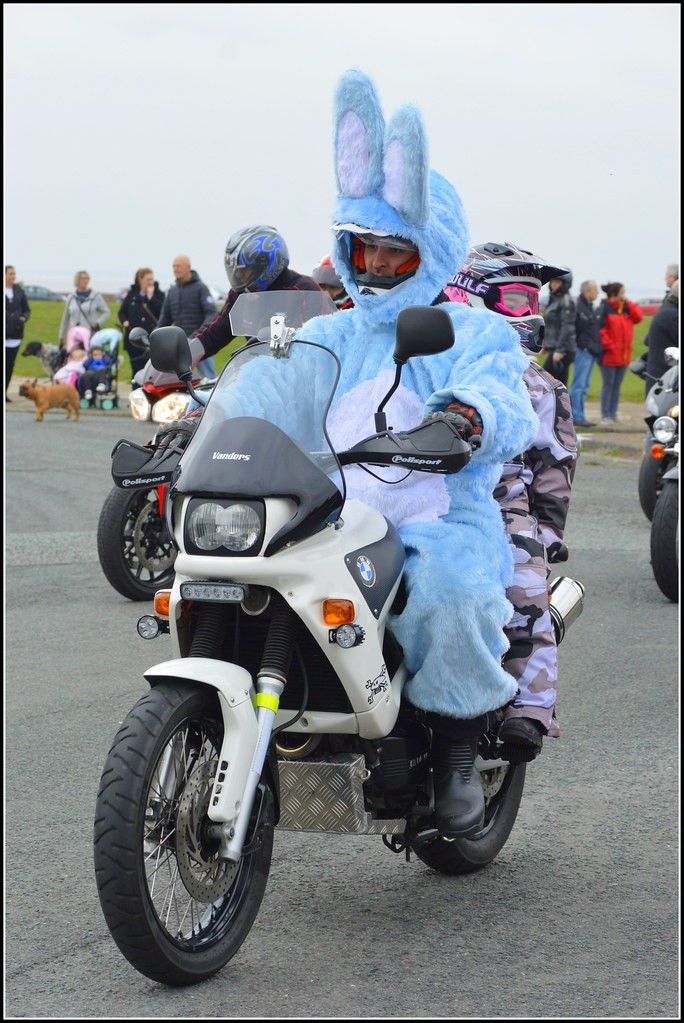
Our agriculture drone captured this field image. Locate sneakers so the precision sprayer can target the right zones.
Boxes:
[500,717,544,747]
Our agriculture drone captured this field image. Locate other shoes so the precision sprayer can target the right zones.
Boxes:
[96,383,106,391]
[599,417,614,425]
[85,389,92,399]
[573,420,595,427]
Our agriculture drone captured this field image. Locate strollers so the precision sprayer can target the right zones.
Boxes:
[73,324,124,410]
[51,325,90,385]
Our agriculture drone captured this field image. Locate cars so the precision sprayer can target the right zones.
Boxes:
[24,285,66,302]
[634,295,665,316]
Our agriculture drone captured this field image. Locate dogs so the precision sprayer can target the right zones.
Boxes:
[18,377,80,422]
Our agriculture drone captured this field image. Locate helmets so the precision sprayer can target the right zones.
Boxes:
[461,244,568,327]
[225,226,288,294]
[350,223,420,296]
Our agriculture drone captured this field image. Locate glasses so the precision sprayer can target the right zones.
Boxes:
[488,283,540,318]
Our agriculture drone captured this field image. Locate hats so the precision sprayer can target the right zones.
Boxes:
[351,231,418,251]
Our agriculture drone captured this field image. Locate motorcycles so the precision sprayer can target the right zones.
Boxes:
[98,326,215,601]
[629,347,679,522]
[92,289,585,988]
[650,388,680,602]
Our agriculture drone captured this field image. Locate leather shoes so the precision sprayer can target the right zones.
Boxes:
[433,734,484,837]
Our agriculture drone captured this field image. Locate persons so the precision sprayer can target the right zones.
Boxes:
[118,268,164,391]
[5,266,31,401]
[644,263,681,452]
[182,67,541,833]
[443,240,575,761]
[154,255,217,379]
[53,270,114,399]
[313,256,354,310]
[541,267,643,428]
[190,224,338,362]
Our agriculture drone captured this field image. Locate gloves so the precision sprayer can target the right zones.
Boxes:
[154,405,204,458]
[421,398,483,451]
